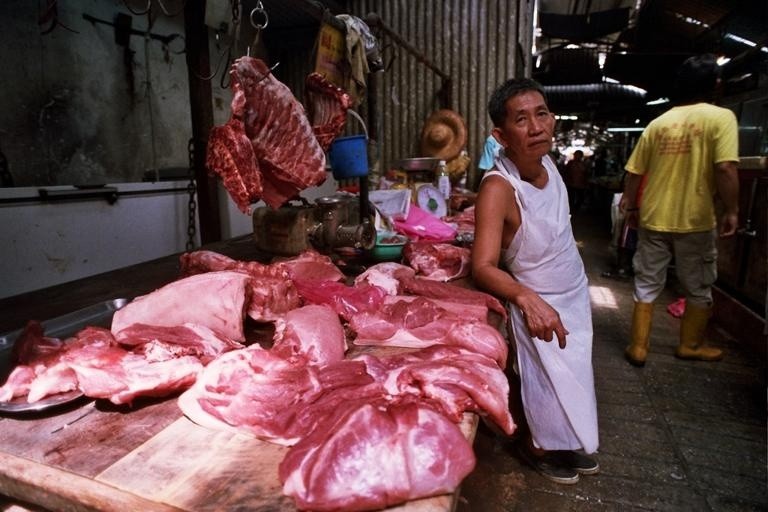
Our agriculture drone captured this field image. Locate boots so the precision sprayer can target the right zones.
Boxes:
[624,301,656,368]
[677,300,725,362]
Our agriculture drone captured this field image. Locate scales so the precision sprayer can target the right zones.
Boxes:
[388,156,449,220]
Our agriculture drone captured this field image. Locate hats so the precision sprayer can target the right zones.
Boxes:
[422,110,468,164]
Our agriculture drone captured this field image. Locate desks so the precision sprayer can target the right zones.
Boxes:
[0,265,504,512]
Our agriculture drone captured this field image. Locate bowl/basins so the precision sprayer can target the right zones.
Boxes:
[372,232,407,258]
[401,157,442,170]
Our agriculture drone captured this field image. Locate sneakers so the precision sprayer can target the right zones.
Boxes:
[559,450,600,476]
[519,442,580,485]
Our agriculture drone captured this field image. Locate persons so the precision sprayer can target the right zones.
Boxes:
[553,149,606,224]
[470,75,602,485]
[478,132,504,180]
[618,53,739,365]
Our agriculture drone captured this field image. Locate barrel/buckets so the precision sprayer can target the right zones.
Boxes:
[329,107,369,180]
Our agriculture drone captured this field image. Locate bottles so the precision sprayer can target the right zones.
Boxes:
[436,161,450,199]
